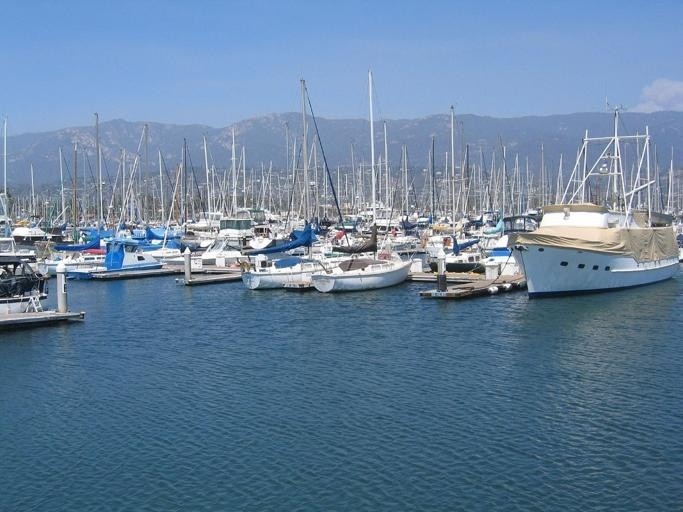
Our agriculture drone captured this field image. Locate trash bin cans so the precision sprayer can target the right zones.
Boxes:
[437,275,446,292]
